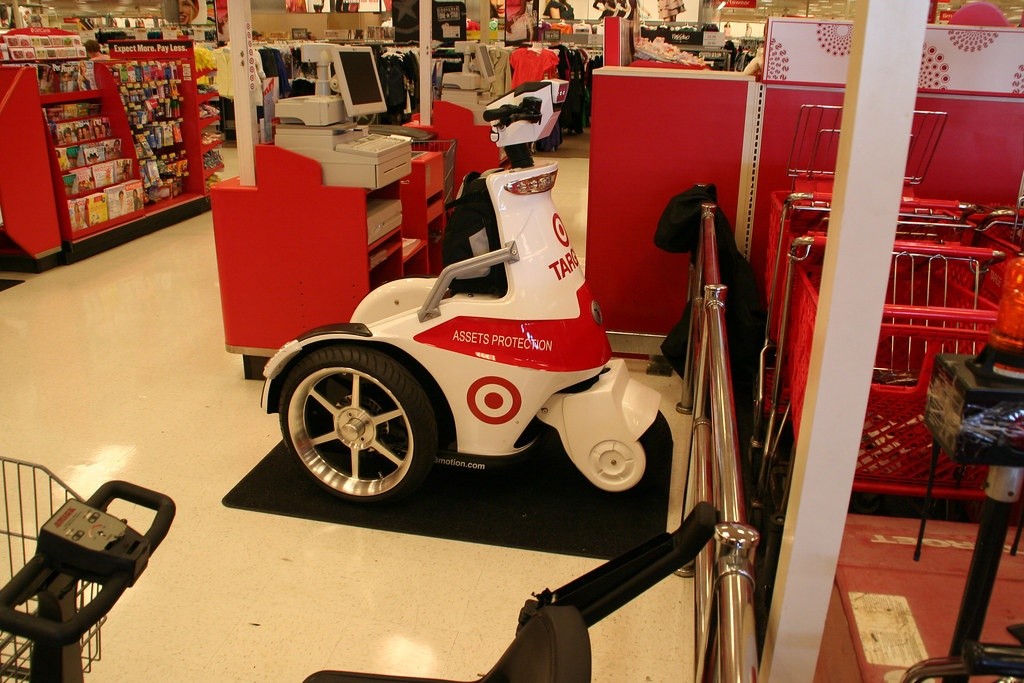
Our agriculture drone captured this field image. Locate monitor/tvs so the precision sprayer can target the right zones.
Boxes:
[333,46,387,117]
[475,43,496,82]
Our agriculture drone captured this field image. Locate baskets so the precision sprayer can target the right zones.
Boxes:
[0,455,108,683]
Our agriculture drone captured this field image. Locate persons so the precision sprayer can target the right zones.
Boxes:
[744,24,767,73]
[85,39,110,61]
[179,0,199,26]
[724,23,753,39]
[489,1,686,46]
[724,40,737,71]
[312,1,361,13]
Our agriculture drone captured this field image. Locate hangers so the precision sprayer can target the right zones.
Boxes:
[194,18,697,62]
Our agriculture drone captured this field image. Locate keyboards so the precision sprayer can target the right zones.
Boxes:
[337,134,409,158]
[479,97,498,105]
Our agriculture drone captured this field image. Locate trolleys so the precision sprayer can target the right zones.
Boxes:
[259,76,664,510]
[749,101,1024,517]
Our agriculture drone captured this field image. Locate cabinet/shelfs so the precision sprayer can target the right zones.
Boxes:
[0,40,448,380]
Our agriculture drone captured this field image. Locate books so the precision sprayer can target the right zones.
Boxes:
[33,62,145,233]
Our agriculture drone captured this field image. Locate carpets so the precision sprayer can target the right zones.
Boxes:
[220,434,675,560]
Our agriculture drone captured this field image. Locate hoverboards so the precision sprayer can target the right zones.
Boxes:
[895,258,1024,683]
[2,455,592,683]
